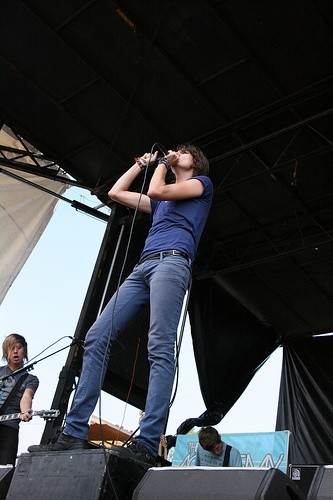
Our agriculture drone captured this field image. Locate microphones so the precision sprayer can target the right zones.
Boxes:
[156,142,169,156]
[68,336,85,348]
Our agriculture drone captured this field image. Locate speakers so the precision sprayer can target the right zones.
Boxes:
[133,464,333,500]
[0,467,15,500]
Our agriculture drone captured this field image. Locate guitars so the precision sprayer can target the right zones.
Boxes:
[0,408,61,422]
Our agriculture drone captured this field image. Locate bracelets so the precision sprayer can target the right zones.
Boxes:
[133,158,146,169]
[159,157,171,168]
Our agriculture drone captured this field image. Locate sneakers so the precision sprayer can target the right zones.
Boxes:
[111,442,155,466]
[26,427,87,453]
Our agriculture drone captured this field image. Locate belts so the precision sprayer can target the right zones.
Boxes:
[149,250,188,262]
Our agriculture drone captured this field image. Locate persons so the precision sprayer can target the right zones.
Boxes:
[27,143,215,467]
[0,333,39,466]
[196,426,243,468]
